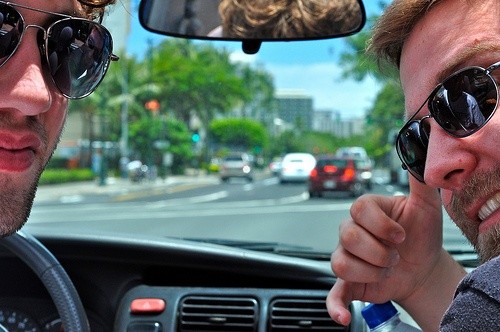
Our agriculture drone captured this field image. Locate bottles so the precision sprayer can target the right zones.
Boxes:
[361,300,422,332]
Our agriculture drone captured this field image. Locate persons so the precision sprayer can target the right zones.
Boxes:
[1,0,114,239]
[432,74,488,131]
[48,22,105,97]
[325,0,500,332]
[209,0,366,41]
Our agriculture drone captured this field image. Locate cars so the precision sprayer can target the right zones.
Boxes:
[308,154,373,199]
[280,153,318,183]
[218,152,254,183]
[269,156,283,177]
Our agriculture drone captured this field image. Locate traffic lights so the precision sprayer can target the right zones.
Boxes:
[142,97,159,110]
[191,130,200,142]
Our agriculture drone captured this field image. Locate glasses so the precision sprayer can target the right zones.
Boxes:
[396,60,500,185]
[1,0,120,102]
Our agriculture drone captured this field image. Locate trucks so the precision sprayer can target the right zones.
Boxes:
[334,145,375,190]
